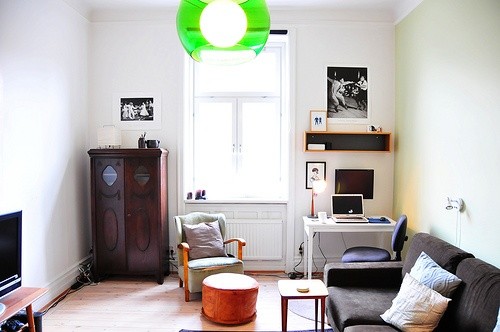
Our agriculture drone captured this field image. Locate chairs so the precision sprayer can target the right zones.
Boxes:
[342,215,408,262]
[173,212,246,302]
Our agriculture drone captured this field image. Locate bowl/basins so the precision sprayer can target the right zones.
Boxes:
[145,140,160,149]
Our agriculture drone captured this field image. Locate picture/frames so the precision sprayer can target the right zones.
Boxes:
[112,92,162,131]
[306,161,326,190]
[323,65,372,124]
[310,109,326,131]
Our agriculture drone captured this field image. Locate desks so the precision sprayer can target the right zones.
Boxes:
[302,216,397,279]
[0,286,48,332]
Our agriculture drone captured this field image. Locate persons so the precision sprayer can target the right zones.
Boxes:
[122,100,153,120]
[355,76,367,110]
[309,167,320,184]
[327,76,355,112]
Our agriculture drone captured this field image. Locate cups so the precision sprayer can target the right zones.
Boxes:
[318,211,326,222]
[138,137,144,148]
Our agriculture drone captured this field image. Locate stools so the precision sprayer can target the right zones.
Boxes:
[278,279,328,332]
[202,272,259,326]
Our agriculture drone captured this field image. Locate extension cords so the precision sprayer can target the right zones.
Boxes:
[281,274,322,277]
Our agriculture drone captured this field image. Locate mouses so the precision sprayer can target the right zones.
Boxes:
[380,217,386,221]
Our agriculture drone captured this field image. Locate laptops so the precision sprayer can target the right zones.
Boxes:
[331,193,369,223]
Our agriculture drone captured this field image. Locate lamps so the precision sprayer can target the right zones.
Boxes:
[308,180,326,218]
[445,196,465,248]
[175,0,270,62]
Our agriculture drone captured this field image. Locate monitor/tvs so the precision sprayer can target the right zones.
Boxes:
[0,210,22,315]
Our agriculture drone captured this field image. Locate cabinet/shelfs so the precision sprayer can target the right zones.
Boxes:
[303,130,393,153]
[86,150,169,284]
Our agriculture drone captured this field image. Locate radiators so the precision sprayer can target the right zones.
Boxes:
[226,218,283,261]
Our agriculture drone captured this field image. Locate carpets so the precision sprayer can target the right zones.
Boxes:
[178,328,336,332]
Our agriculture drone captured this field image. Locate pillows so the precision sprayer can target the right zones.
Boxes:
[182,220,227,259]
[410,251,461,298]
[380,273,451,332]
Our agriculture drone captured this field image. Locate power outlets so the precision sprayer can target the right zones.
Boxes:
[169,247,176,262]
[298,247,304,256]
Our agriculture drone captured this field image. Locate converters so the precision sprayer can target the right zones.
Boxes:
[288,272,297,279]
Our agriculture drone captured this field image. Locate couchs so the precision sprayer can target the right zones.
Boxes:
[325,234,500,332]
